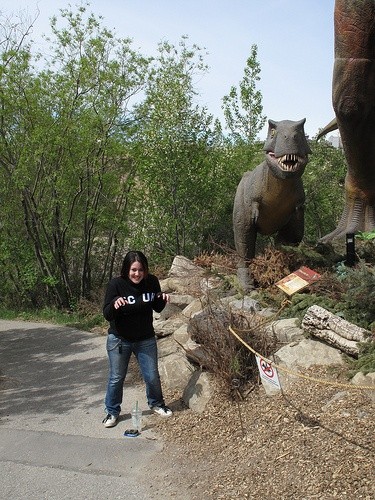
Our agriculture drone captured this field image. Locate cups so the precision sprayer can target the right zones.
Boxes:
[130,401,142,432]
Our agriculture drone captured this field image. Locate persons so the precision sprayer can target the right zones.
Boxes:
[102,251,173,427]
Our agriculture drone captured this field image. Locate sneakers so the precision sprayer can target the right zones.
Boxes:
[152,406,173,417]
[102,412,119,427]
